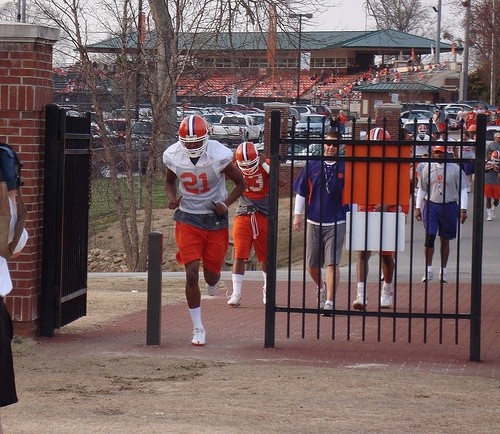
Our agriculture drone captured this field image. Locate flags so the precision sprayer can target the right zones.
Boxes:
[431,47,434,58]
[452,44,456,58]
[412,49,415,64]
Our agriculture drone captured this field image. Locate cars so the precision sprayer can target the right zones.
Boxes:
[60,99,369,164]
[399,99,500,158]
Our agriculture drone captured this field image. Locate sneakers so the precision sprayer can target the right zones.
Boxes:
[263,290,266,305]
[381,291,392,308]
[206,282,219,296]
[322,303,333,316]
[317,282,326,309]
[352,296,368,311]
[191,327,205,345]
[226,290,241,305]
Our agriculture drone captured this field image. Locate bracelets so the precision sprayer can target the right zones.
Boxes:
[221,202,228,210]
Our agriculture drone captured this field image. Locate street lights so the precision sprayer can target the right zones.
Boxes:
[288,12,314,105]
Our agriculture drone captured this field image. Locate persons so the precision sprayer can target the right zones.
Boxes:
[414,106,500,284]
[292,131,353,318]
[162,115,246,346]
[352,128,409,309]
[329,115,338,132]
[227,142,285,306]
[335,101,362,110]
[337,110,346,134]
[0,143,27,408]
[196,58,449,99]
[52,60,112,94]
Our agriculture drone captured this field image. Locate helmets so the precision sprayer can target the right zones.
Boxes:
[467,125,476,131]
[235,142,259,175]
[180,115,208,158]
[365,128,390,140]
[418,125,427,138]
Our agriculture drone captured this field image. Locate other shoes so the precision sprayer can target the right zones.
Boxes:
[422,271,434,282]
[468,186,472,193]
[487,215,492,220]
[439,273,447,283]
[491,206,496,218]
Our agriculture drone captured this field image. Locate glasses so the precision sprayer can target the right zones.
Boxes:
[435,151,444,154]
[326,144,337,148]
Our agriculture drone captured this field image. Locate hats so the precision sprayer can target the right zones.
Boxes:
[434,109,439,112]
[434,146,444,152]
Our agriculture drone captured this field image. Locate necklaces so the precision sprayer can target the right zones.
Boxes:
[436,169,443,196]
